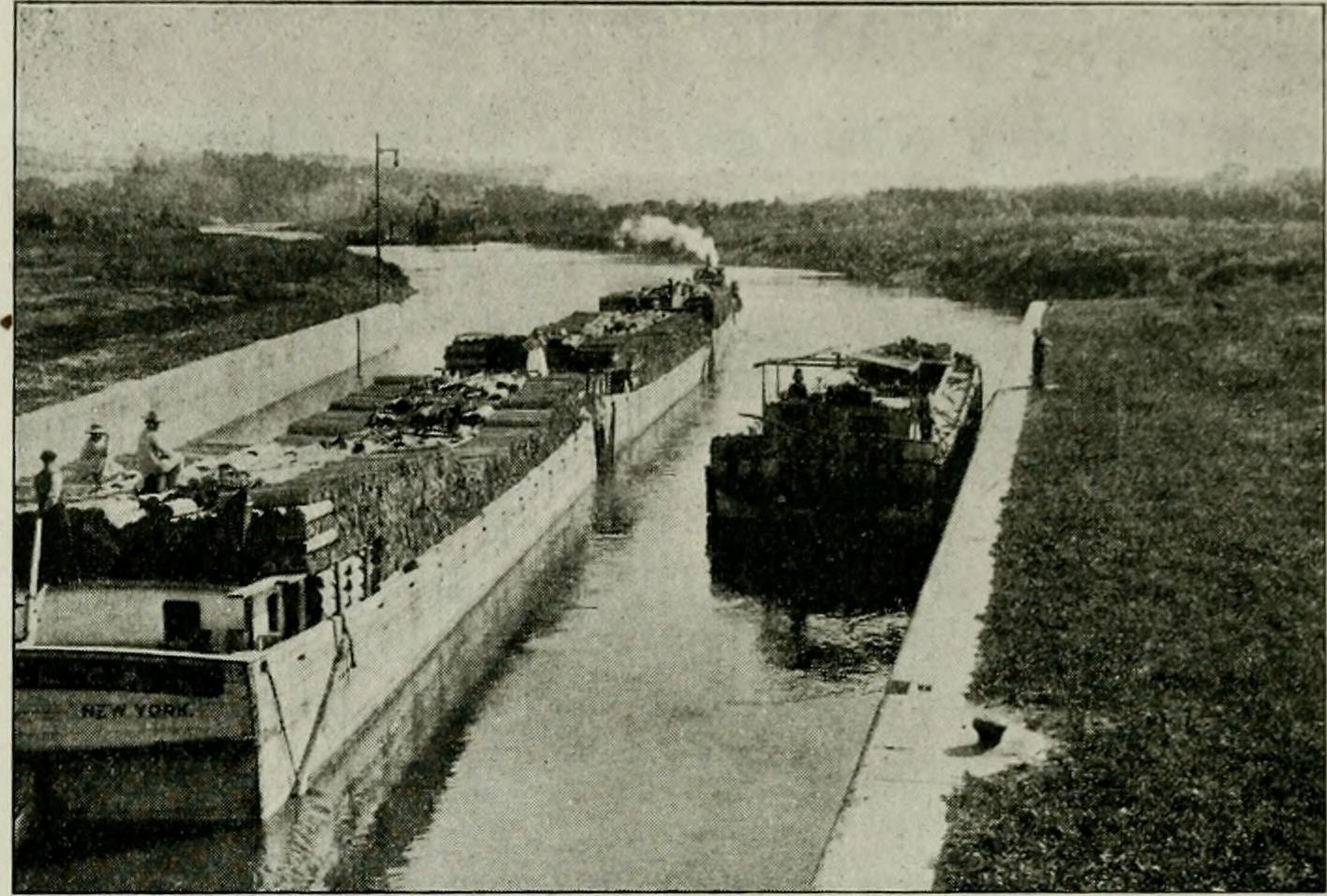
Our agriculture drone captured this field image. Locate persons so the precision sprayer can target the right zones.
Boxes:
[788,369,808,396]
[34,450,77,581]
[137,409,183,483]
[1032,328,1054,388]
[80,423,130,488]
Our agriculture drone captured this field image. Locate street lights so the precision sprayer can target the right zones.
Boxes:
[375,131,400,306]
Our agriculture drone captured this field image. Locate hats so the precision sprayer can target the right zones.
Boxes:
[140,410,165,421]
[40,450,57,462]
[84,424,106,433]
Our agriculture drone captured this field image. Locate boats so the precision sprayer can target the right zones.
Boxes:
[434,250,744,465]
[703,332,986,608]
[13,370,599,835]
